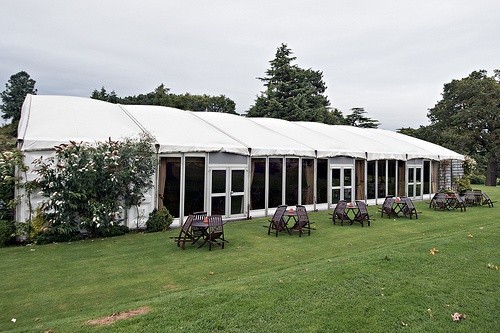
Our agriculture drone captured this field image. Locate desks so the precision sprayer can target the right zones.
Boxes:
[447,196,457,208]
[283,211,297,226]
[346,205,358,217]
[394,200,406,215]
[192,219,228,247]
[464,192,483,206]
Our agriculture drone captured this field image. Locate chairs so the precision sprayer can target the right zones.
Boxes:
[426,190,498,211]
[170,214,230,251]
[377,195,423,219]
[328,201,375,227]
[263,205,316,237]
